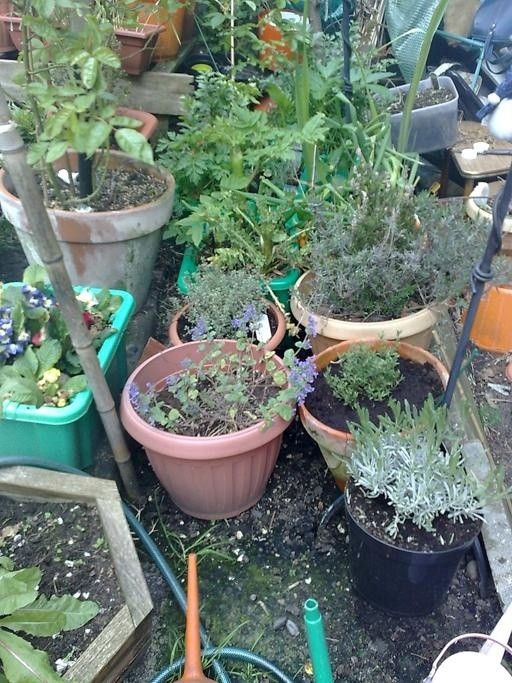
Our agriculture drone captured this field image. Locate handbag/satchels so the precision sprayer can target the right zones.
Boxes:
[470,0,511,75]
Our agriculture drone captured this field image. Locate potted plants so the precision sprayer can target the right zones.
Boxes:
[1,2,511,683]
